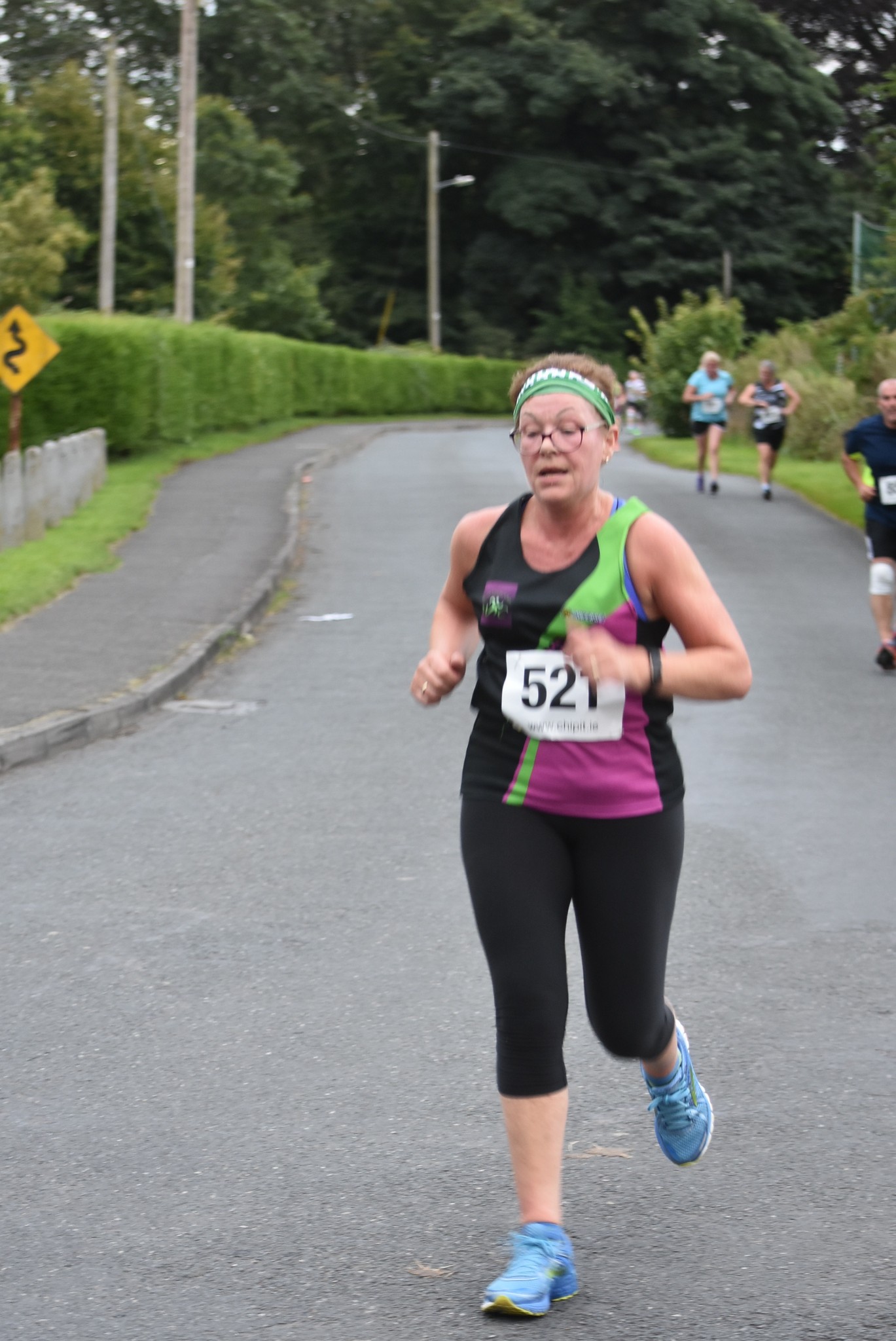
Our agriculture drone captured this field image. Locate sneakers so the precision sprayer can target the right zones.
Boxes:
[480,1222,579,1317]
[643,1020,715,1168]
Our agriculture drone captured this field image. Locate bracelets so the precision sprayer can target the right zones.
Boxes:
[644,645,663,696]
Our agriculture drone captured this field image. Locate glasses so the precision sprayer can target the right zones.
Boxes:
[510,420,606,456]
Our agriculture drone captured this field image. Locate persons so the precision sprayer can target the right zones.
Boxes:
[608,368,649,435]
[409,353,753,1319]
[840,378,896,672]
[738,360,803,500]
[680,353,738,495]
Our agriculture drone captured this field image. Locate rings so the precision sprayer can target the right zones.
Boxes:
[420,680,429,696]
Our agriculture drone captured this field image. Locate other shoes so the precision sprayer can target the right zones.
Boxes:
[877,642,896,670]
[763,489,772,501]
[711,483,718,493]
[697,477,704,491]
